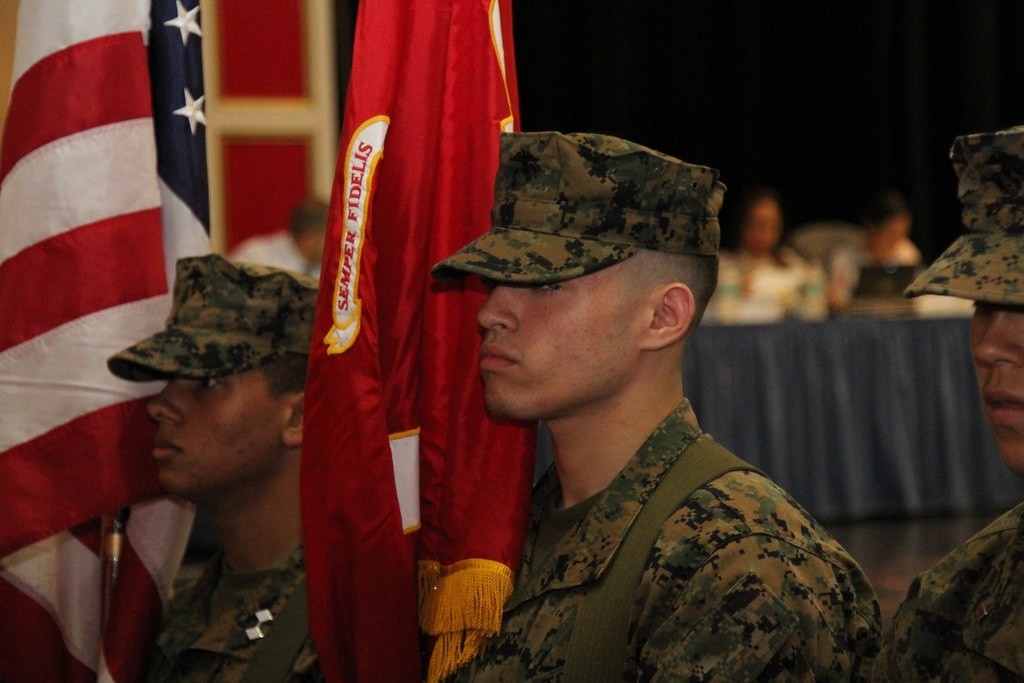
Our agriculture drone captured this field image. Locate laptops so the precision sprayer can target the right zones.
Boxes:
[853,263,915,306]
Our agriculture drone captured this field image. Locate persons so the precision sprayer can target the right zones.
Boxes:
[229,191,330,276]
[700,195,920,329]
[108,254,330,683]
[871,124,1023,683]
[431,133,883,683]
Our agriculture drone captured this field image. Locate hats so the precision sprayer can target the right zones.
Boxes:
[107,253,320,382]
[430,131,728,283]
[902,125,1024,306]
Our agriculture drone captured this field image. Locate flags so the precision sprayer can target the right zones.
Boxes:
[0,0,210,682]
[301,0,539,682]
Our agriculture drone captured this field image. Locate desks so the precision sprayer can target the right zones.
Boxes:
[682,300,1023,523]
